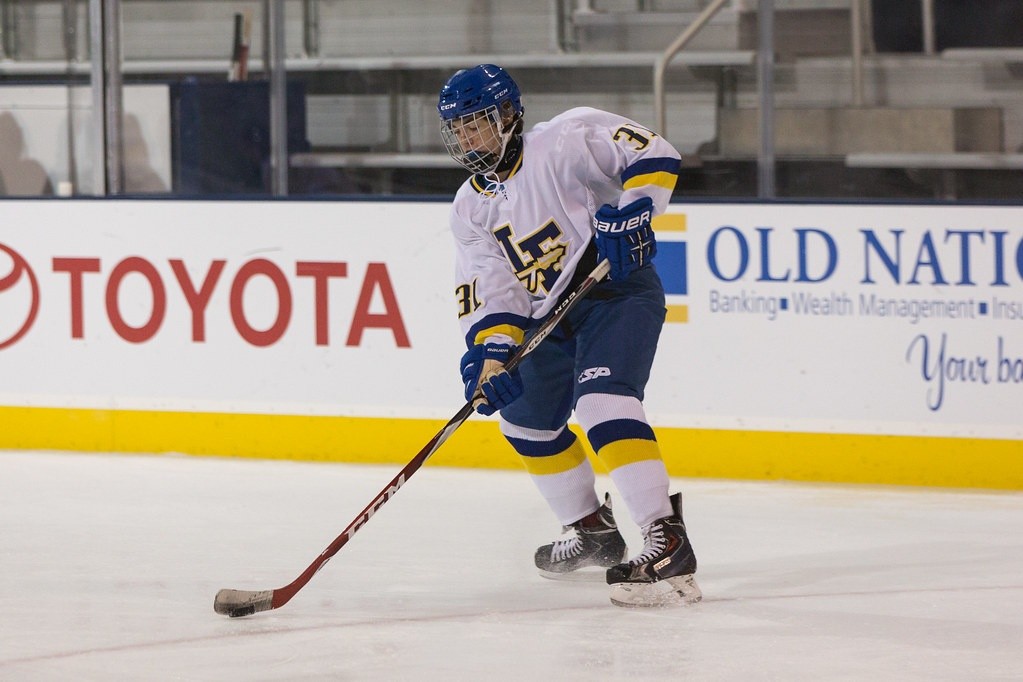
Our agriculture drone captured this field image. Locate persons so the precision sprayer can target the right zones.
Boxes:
[438,64,703,607]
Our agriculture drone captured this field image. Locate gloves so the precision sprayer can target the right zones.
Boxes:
[460,341,523,416]
[592,196,657,280]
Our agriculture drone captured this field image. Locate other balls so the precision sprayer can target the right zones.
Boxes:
[228,603,255,618]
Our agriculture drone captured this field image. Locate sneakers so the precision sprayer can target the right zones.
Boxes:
[534,492,629,582]
[607,492,702,608]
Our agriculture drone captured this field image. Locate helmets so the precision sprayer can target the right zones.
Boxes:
[436,63,524,176]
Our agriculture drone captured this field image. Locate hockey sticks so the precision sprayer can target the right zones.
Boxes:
[212,256,611,616]
